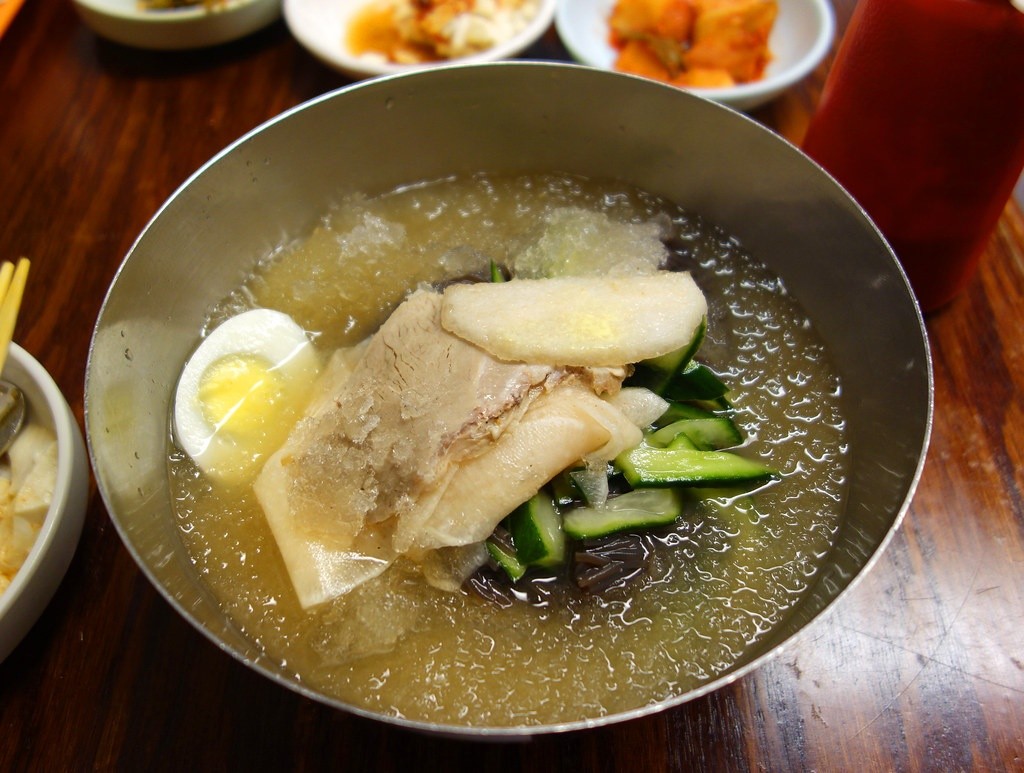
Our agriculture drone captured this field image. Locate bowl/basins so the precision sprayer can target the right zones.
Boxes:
[82,62,938,737]
[555,0,836,113]
[282,0,554,80]
[72,0,280,49]
[0,337,89,662]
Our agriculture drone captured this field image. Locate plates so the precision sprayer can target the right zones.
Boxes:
[0,0,25,40]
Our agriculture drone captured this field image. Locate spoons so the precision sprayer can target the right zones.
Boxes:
[0,377,27,458]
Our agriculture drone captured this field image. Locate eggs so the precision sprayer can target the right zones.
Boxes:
[174,309,322,483]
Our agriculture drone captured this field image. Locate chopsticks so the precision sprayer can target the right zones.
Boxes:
[0,257,32,375]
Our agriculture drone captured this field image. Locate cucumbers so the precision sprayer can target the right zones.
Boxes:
[478,257,778,583]
